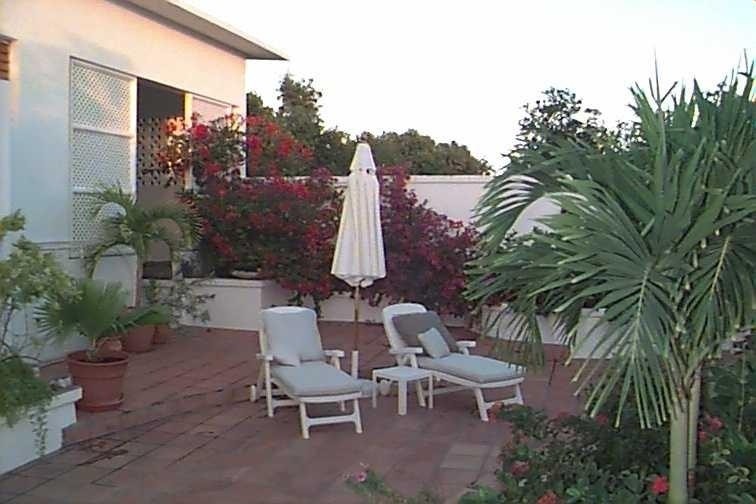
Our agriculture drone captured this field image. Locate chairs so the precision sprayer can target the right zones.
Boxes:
[381,303,528,423]
[249,305,365,439]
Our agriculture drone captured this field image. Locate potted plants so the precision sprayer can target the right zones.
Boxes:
[79,177,204,353]
[31,276,173,414]
[145,267,217,343]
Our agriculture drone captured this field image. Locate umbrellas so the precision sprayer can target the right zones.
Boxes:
[329,143,388,350]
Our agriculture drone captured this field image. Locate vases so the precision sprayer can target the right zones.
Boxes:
[97,337,121,352]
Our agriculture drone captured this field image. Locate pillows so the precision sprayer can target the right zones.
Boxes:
[392,310,459,357]
[416,327,451,360]
[260,308,323,367]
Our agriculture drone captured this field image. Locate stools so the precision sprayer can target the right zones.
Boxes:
[372,366,434,416]
